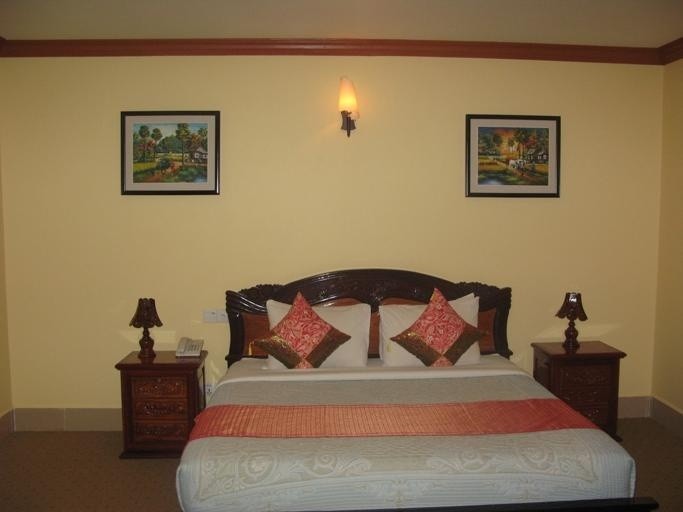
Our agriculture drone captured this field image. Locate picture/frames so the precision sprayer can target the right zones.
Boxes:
[119,111,220,195]
[466,114,561,198]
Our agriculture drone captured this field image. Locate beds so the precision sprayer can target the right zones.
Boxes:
[173,269,636,511]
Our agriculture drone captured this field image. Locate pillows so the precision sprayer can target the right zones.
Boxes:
[252,287,490,372]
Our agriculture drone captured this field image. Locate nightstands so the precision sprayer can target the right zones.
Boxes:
[115,351,208,458]
[531,342,627,436]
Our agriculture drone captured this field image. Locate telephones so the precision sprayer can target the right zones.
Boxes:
[175,336,204,357]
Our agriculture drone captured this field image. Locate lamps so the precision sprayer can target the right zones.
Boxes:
[338,75,358,138]
[555,293,588,347]
[129,297,163,358]
